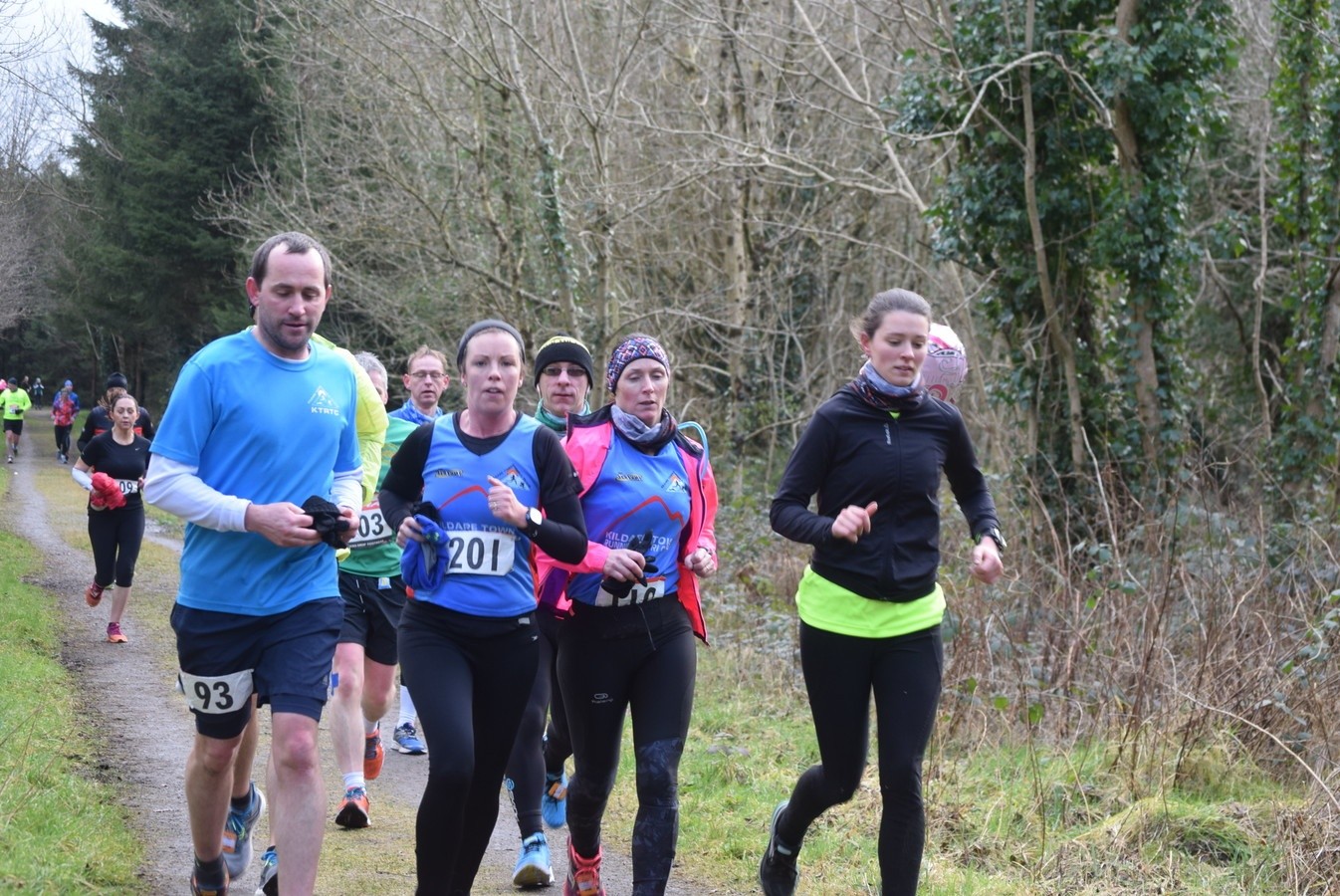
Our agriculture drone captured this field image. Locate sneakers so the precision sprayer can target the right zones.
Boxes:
[86,582,104,607]
[221,787,267,880]
[8,457,13,463]
[511,832,555,887]
[334,786,371,827]
[13,446,17,455]
[759,800,804,895]
[563,835,606,896]
[542,734,568,828]
[363,720,384,779]
[189,858,229,896]
[391,723,427,754]
[107,623,127,643]
[254,847,278,896]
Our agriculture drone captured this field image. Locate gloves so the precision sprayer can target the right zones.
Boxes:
[601,530,659,598]
[300,495,349,549]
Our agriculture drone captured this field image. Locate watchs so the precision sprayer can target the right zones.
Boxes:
[980,527,1007,561]
[516,507,542,533]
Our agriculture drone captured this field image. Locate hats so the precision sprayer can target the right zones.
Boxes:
[534,334,593,389]
[8,378,17,385]
[65,380,73,387]
[107,372,127,390]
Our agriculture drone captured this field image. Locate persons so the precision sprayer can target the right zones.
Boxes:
[209,293,387,896]
[77,373,157,457]
[757,288,1007,896]
[0,374,80,464]
[140,231,361,896]
[371,320,589,896]
[386,347,450,753]
[531,334,718,896]
[71,391,153,643]
[500,335,593,891]
[330,349,420,828]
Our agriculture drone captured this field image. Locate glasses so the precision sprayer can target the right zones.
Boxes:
[543,367,585,376]
[409,372,445,381]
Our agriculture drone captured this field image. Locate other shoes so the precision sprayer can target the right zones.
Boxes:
[62,458,67,464]
[57,451,61,459]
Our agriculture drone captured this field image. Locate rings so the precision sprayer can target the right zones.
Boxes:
[707,566,710,571]
[493,502,497,510]
